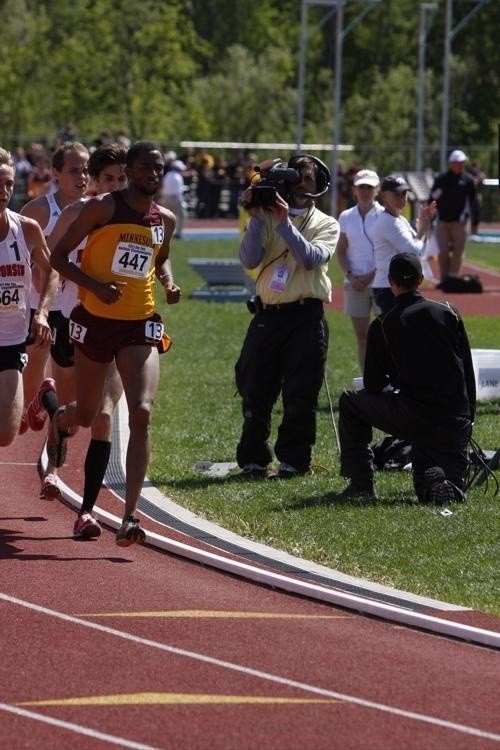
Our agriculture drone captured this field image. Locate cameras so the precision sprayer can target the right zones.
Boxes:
[240,157,300,210]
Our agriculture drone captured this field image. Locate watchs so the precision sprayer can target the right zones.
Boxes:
[346,270,353,277]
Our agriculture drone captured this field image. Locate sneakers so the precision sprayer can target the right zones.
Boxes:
[228,464,268,482]
[270,463,304,481]
[115,514,145,547]
[46,409,69,467]
[326,482,374,504]
[423,466,455,506]
[28,378,56,432]
[37,474,62,502]
[73,513,102,537]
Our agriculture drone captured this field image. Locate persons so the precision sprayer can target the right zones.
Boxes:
[374,173,437,309]
[471,163,485,206]
[333,168,383,377]
[337,252,477,509]
[162,147,249,234]
[30,146,127,539]
[9,125,131,183]
[0,146,60,450]
[22,142,90,501]
[340,162,360,212]
[428,149,481,281]
[45,142,181,546]
[235,156,341,479]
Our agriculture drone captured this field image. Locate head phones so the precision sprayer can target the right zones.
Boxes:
[287,152,334,199]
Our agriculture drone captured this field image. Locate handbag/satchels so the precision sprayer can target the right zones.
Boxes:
[437,272,483,294]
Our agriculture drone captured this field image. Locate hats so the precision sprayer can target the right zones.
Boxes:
[450,150,466,163]
[354,169,380,188]
[382,175,413,193]
[388,250,422,283]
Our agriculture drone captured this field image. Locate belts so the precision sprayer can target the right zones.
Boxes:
[262,298,314,311]
